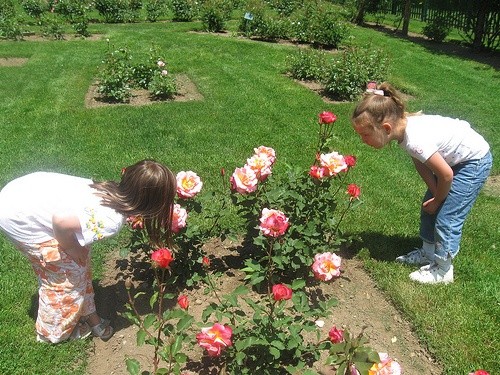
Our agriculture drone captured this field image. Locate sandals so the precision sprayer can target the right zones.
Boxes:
[36,324,92,343]
[79,311,113,339]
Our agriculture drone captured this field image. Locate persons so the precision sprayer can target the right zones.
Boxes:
[0,159,177,345]
[352,83,493,284]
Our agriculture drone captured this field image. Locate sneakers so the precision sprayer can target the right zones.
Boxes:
[395,247,433,266]
[408,264,453,285]
